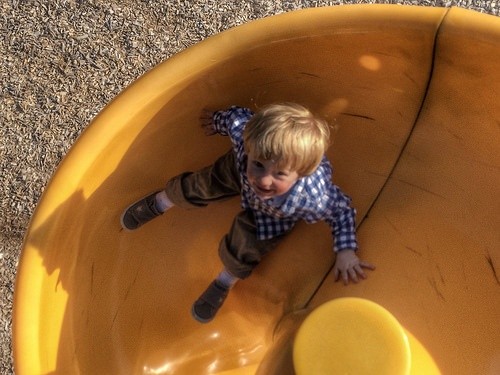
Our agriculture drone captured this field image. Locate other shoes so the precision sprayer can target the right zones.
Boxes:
[120,188,167,232]
[190,280,231,324]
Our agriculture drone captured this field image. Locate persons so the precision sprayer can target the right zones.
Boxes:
[121,102,376,323]
